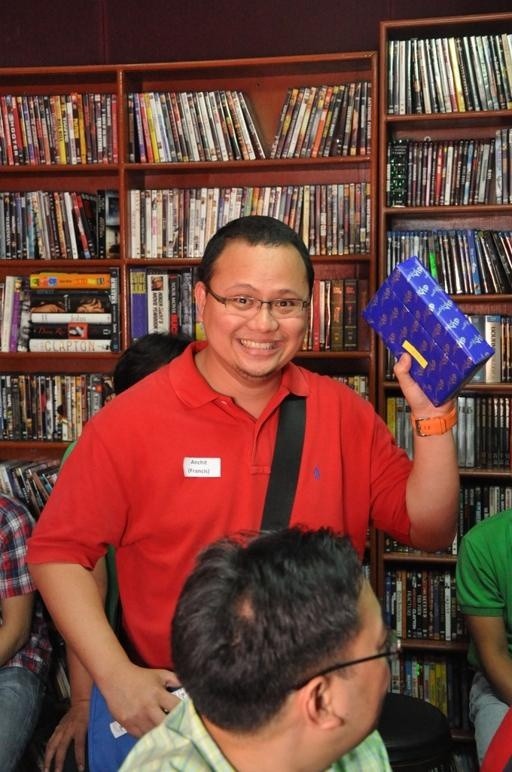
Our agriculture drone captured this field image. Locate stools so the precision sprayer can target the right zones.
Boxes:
[379,690,460,772]
[38,696,69,756]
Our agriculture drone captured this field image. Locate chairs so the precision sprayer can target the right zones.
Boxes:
[88,679,139,772]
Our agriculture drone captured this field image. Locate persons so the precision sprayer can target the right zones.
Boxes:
[117,524,394,770]
[0,493,56,770]
[44,334,201,771]
[23,218,465,740]
[458,504,511,769]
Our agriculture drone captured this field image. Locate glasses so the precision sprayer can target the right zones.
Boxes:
[204,287,310,317]
[293,635,402,689]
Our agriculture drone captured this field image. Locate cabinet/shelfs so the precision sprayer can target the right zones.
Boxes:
[2,50,376,601]
[378,12,512,772]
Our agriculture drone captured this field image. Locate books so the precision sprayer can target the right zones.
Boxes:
[381,569,463,717]
[126,81,371,162]
[129,268,371,351]
[387,32,512,116]
[128,183,371,259]
[322,375,370,403]
[0,458,61,520]
[387,228,511,383]
[52,652,73,712]
[2,92,121,442]
[384,486,510,555]
[387,394,511,471]
[385,129,512,206]
